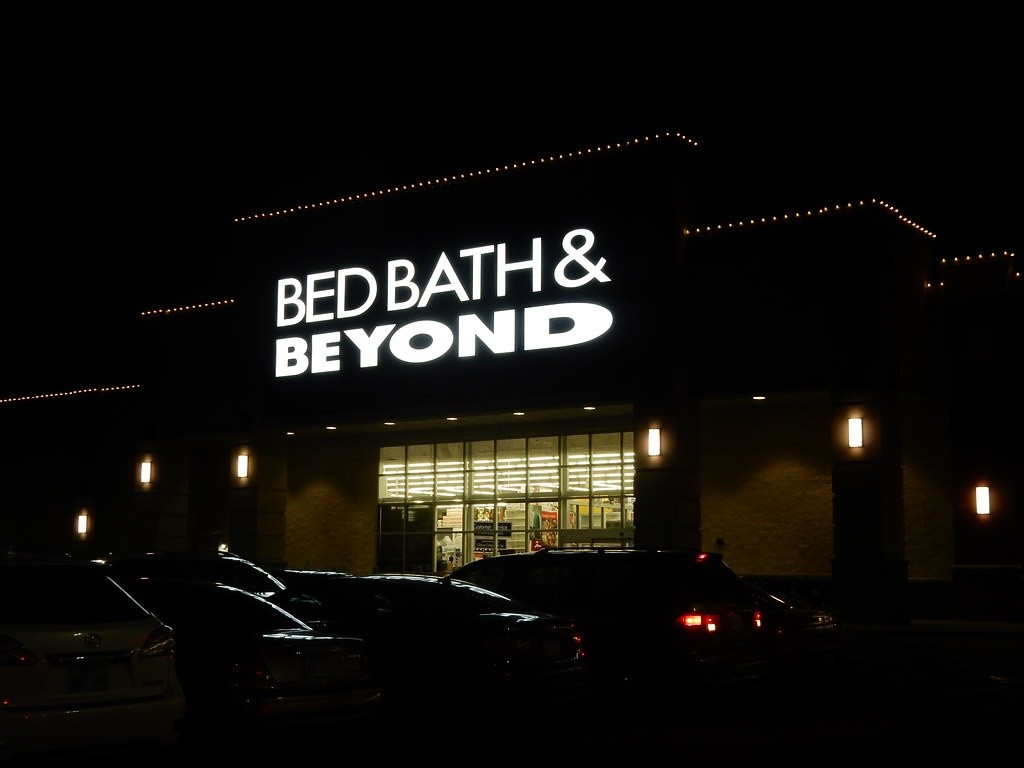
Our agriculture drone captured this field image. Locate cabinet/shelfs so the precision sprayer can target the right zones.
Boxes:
[581,513,628,547]
[502,509,530,553]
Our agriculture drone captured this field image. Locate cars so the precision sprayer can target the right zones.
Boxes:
[284,575,590,738]
[740,578,839,655]
[99,549,341,635]
[116,573,385,746]
[0,553,189,768]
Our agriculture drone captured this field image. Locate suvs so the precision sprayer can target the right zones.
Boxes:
[442,544,761,712]
